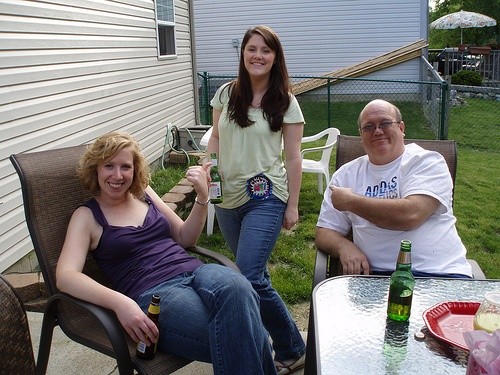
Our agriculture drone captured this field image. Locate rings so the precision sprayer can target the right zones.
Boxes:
[294,224,296,226]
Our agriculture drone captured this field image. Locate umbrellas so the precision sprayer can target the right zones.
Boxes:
[429,9,497,44]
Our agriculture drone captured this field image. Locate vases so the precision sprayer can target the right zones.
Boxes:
[471,47,491,54]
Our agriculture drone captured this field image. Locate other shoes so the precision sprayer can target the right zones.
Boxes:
[273,351,306,375]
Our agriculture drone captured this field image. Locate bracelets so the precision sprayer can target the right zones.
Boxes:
[195,196,210,206]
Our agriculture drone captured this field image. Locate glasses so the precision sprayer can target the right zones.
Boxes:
[360,119,400,133]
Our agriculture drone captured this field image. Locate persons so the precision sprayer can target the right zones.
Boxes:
[208,26,306,375]
[314,98,473,278]
[56,132,275,375]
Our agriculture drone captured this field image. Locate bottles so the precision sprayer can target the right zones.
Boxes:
[209,153,223,204]
[386,240,415,322]
[473,291,500,335]
[136,294,161,361]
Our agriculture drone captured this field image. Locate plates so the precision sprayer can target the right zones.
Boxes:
[422,300,481,353]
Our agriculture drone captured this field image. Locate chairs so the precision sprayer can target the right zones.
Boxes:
[304,134,457,375]
[282,128,340,193]
[462,54,483,68]
[10,143,243,375]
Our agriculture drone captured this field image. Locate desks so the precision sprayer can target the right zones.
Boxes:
[304,275,500,375]
